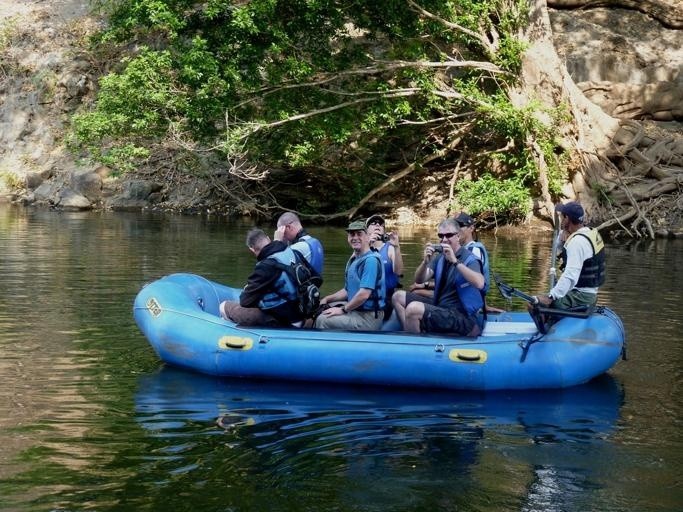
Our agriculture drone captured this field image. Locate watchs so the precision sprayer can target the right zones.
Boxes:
[424,281,428,289]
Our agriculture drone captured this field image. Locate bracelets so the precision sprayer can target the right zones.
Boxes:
[340,305,349,314]
[453,259,461,268]
[546,292,553,300]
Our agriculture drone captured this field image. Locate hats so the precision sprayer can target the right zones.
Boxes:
[345,215,385,233]
[455,213,474,226]
[556,202,584,222]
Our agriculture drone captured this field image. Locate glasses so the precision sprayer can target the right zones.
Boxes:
[438,233,457,238]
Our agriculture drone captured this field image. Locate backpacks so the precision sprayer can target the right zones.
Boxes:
[261,251,320,316]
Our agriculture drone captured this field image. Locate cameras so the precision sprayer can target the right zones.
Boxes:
[377,233,391,243]
[427,243,443,254]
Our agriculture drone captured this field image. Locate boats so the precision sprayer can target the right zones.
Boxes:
[131,273,626,393]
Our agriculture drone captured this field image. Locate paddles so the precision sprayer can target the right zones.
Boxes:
[493,274,535,303]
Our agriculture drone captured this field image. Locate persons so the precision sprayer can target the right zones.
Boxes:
[364,214,403,321]
[217,228,325,329]
[391,219,485,339]
[453,214,491,323]
[273,213,326,287]
[525,202,607,335]
[314,219,387,334]
